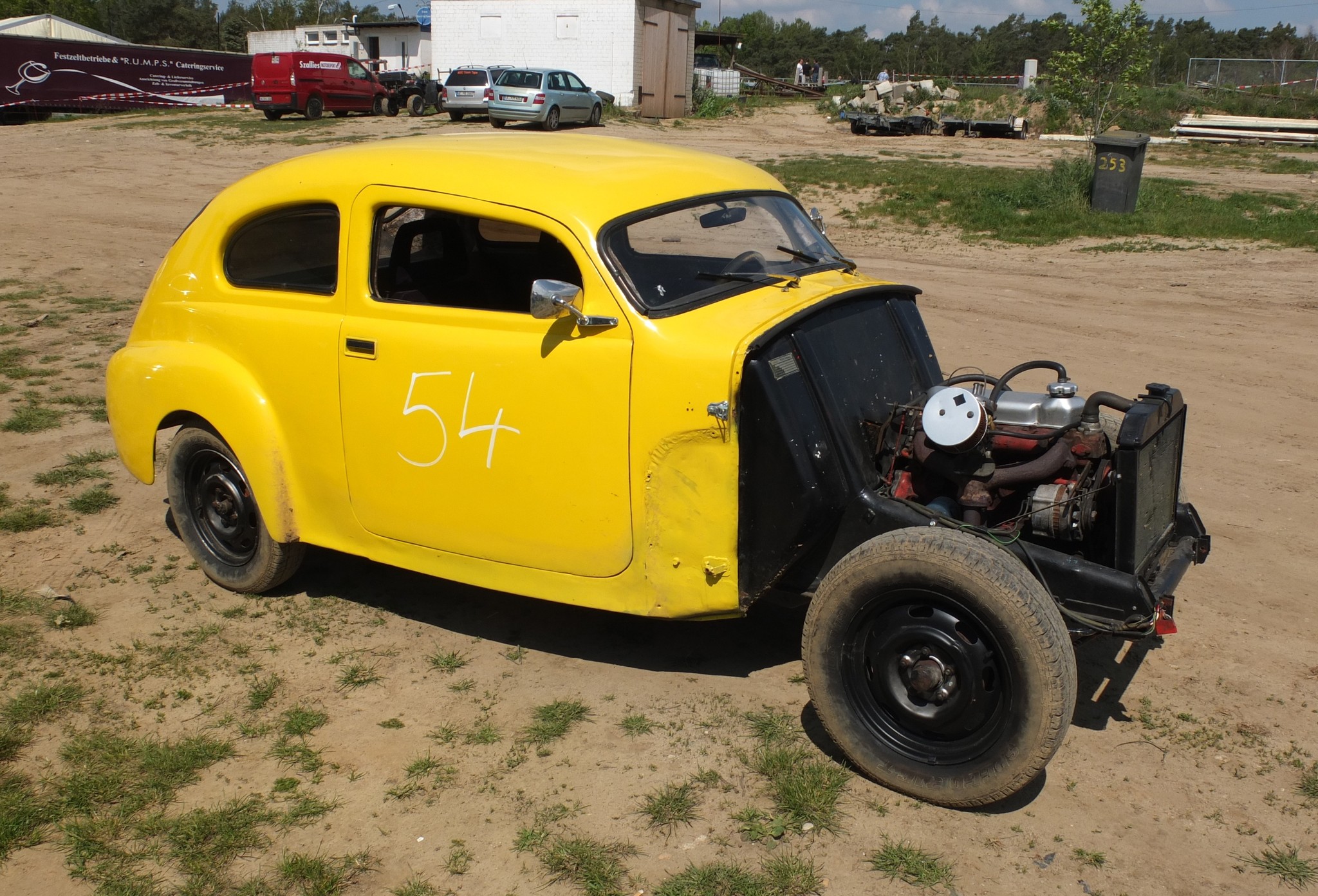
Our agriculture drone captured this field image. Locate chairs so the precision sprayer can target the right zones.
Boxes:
[553,77,559,86]
[524,76,537,86]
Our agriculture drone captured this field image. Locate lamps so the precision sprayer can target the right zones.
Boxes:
[735,38,743,49]
[388,3,405,21]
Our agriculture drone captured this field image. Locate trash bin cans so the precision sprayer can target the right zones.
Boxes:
[1090,129,1150,212]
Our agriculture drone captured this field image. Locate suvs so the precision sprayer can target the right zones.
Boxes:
[441,65,522,121]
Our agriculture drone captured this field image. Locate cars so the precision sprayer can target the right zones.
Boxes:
[488,68,603,131]
[106,132,1212,807]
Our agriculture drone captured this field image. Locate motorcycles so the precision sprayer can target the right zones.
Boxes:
[378,70,447,117]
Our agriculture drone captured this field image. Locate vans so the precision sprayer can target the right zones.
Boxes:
[252,51,388,121]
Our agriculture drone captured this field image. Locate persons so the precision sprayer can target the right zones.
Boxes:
[835,76,843,83]
[797,58,811,83]
[877,69,889,82]
[811,59,819,83]
